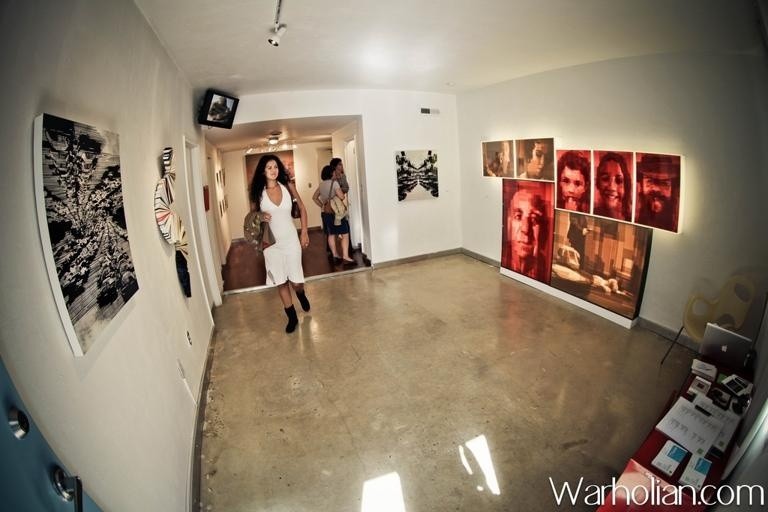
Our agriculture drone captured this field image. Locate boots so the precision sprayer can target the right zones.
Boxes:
[296,290,310,312]
[284,304,298,333]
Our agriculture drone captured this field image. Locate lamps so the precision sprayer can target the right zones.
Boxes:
[267,22,287,48]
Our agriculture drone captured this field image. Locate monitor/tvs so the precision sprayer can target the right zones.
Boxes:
[197,88,239,129]
[697,323,752,372]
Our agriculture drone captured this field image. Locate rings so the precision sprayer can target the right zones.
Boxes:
[305,243,309,247]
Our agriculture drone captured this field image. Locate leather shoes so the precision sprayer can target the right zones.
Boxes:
[327,248,356,264]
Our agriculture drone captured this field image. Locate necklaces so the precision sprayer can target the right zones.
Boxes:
[266,183,279,191]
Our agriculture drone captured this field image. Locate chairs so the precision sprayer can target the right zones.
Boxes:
[660,275,756,364]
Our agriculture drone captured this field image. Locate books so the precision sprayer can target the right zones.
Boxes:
[596,457,703,512]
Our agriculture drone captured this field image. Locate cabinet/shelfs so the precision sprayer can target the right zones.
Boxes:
[595,355,757,511]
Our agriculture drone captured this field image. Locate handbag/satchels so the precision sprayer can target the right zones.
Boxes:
[285,183,300,218]
[324,196,347,214]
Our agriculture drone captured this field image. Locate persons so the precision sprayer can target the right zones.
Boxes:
[327,157,355,256]
[505,188,548,281]
[249,155,313,333]
[636,153,679,232]
[556,151,590,214]
[518,140,553,179]
[568,211,592,271]
[312,165,356,266]
[483,142,512,176]
[595,152,632,224]
